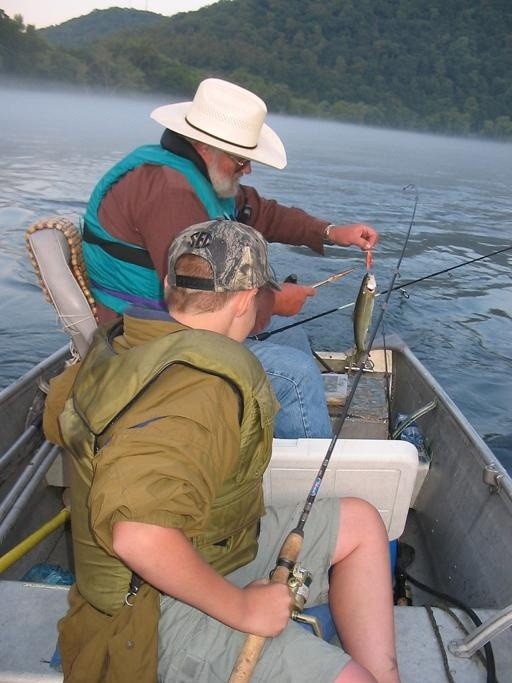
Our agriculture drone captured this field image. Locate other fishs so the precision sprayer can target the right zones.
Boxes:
[352,273,377,364]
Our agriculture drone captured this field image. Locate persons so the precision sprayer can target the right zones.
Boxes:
[79,77,416,573]
[44,217,399,683]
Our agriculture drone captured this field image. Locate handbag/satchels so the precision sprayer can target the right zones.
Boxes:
[58,583,160,683]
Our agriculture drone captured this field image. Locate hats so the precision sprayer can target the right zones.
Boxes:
[168,217,283,292]
[151,78,288,170]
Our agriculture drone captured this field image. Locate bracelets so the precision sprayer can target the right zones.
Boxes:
[322,224,336,245]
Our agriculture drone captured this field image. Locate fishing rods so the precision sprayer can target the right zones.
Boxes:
[229,181,420,680]
[240,247,511,341]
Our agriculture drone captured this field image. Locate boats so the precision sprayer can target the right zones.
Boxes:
[0,332,512,682]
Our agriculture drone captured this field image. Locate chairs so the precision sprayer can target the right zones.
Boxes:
[25,210,101,361]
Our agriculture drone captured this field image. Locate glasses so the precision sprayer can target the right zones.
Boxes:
[228,154,249,171]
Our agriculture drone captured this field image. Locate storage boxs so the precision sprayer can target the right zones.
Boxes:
[263,438,419,579]
